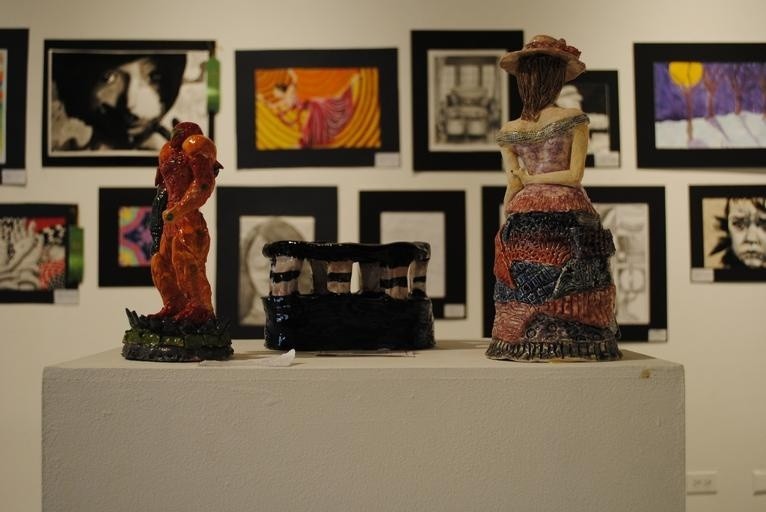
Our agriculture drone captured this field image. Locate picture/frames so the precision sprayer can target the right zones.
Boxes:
[684,180,766,288]
[630,38,766,173]
[355,185,471,325]
[405,27,526,175]
[230,45,404,172]
[508,63,625,171]
[95,183,166,292]
[211,182,343,343]
[39,35,219,169]
[478,182,675,344]
[2,199,88,308]
[0,24,31,189]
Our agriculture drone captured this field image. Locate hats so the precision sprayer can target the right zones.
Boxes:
[499,35,585,81]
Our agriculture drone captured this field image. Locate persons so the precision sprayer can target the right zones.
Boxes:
[256,68,363,149]
[709,197,766,269]
[52,54,187,150]
[485,36,623,363]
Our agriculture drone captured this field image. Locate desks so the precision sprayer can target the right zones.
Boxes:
[41,347,686,512]
